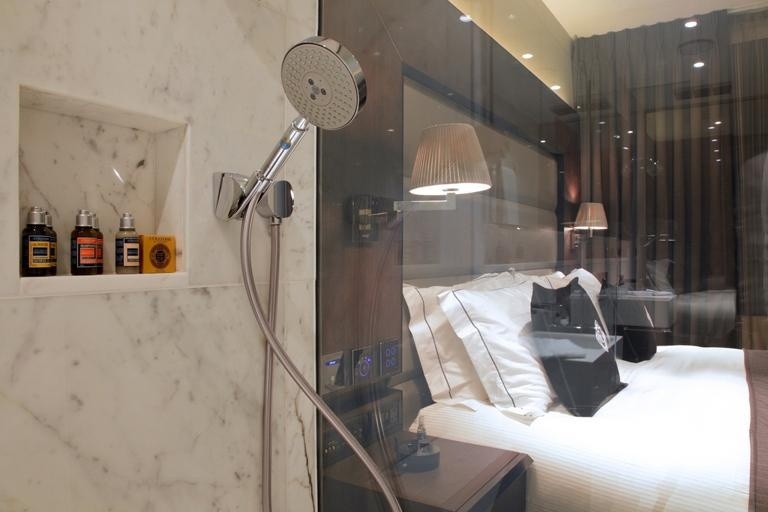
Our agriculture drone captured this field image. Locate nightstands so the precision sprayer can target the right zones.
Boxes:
[318,429,532,512]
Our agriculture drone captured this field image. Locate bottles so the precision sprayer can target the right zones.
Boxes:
[21,205,140,277]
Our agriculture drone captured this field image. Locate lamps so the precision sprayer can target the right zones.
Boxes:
[350,122,494,245]
[571,202,608,251]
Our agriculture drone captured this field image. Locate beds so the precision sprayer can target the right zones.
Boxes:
[418,343,768,512]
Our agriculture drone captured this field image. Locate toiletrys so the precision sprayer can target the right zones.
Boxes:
[20,204,142,276]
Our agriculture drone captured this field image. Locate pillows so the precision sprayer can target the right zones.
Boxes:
[402,267,659,418]
